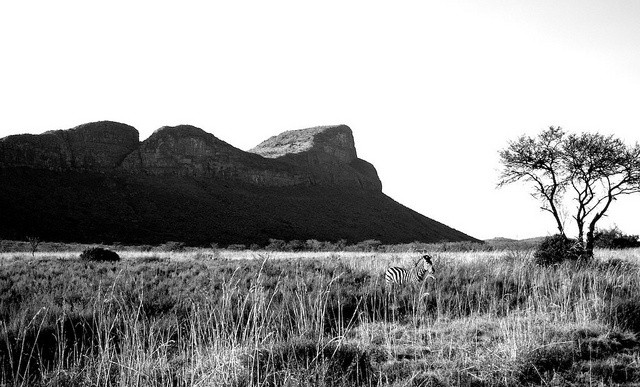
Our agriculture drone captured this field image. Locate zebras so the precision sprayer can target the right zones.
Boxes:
[384,255,435,294]
